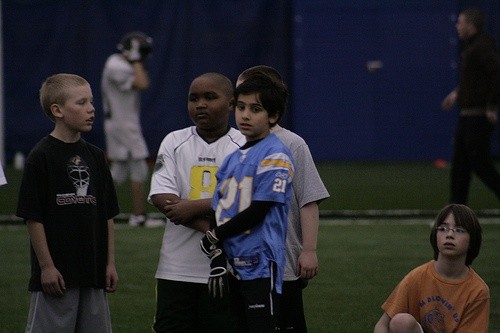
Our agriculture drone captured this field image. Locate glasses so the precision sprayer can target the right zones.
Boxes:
[435,227,466,235]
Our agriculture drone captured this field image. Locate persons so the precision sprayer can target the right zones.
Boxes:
[100,32,166,228]
[440,6,500,203]
[15,74,121,333]
[236,66,330,333]
[201,74,295,333]
[373,203,489,333]
[147,72,247,333]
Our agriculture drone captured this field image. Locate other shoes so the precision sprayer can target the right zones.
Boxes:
[129,213,165,229]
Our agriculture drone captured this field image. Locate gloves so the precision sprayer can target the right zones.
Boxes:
[199,228,219,258]
[123,37,140,62]
[206,249,241,299]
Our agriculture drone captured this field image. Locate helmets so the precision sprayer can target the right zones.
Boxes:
[121,32,153,52]
